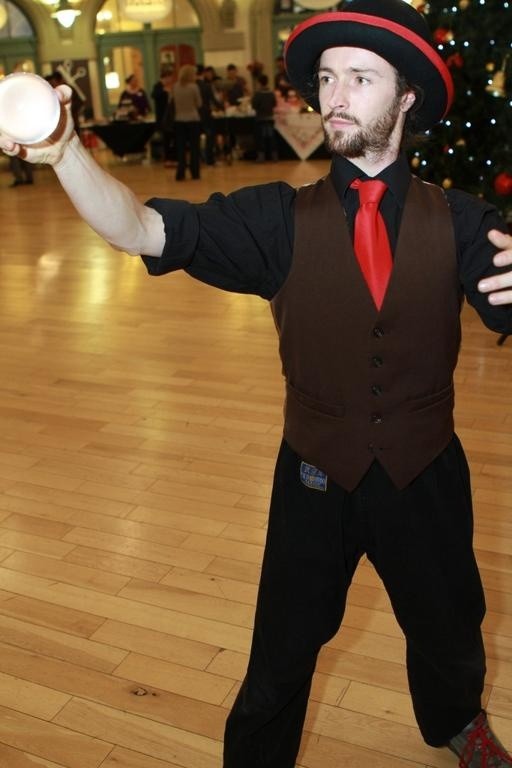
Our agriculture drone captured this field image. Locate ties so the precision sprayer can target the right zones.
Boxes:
[350,178,395,314]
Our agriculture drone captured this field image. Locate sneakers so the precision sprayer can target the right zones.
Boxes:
[451,708,512,768]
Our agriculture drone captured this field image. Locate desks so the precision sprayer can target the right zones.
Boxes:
[88,114,277,168]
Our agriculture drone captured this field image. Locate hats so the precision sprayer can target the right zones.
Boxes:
[281,0,456,137]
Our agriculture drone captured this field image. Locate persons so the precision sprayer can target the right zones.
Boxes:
[0,0,511,767]
[1,55,294,188]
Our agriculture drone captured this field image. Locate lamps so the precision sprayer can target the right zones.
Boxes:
[50,0,81,29]
